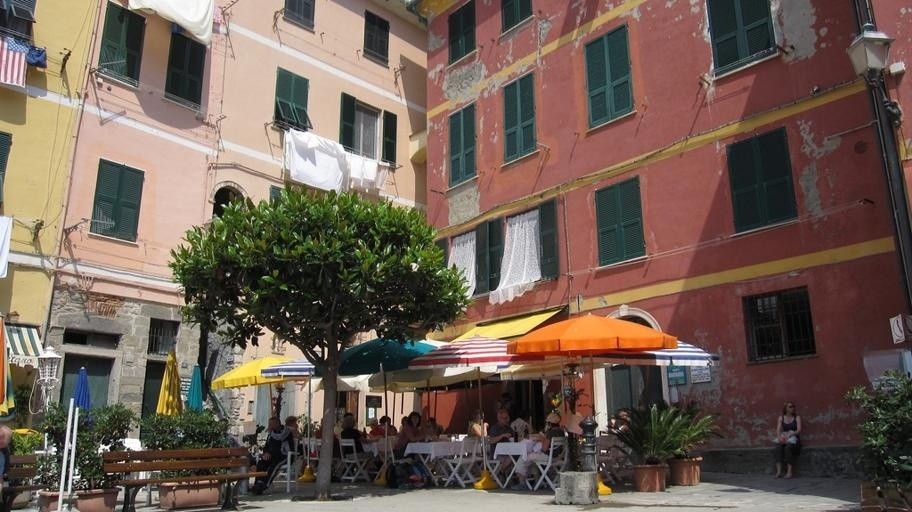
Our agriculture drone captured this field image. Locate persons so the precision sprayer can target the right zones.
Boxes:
[772,401,801,478]
[333,411,444,484]
[468,409,565,491]
[218,415,313,495]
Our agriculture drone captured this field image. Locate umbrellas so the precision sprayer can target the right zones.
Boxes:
[156,347,182,415]
[74,362,92,428]
[187,363,202,412]
[1,314,15,417]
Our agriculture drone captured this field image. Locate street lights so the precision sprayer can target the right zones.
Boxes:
[33,344,63,506]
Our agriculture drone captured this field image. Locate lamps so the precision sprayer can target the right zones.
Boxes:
[846,22,902,121]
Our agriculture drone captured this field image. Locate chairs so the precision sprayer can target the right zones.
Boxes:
[534,437,567,492]
[302,437,319,462]
[859,479,911,512]
[339,440,371,482]
[444,437,479,488]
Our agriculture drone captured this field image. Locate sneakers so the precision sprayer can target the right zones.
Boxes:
[511,483,526,490]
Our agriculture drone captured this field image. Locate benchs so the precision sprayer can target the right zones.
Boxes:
[102,447,268,511]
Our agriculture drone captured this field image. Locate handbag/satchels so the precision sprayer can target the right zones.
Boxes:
[385,462,412,488]
[771,431,798,444]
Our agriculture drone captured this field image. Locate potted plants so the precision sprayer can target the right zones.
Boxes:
[141,408,234,510]
[601,403,691,492]
[39,401,68,512]
[75,403,143,512]
[667,400,722,486]
[8,431,44,508]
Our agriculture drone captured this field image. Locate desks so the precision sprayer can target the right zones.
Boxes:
[375,437,398,480]
[403,441,480,488]
[494,439,538,489]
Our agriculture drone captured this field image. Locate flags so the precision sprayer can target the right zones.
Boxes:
[0,36,30,88]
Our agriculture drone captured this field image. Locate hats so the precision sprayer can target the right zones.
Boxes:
[546,413,561,424]
[343,416,356,429]
